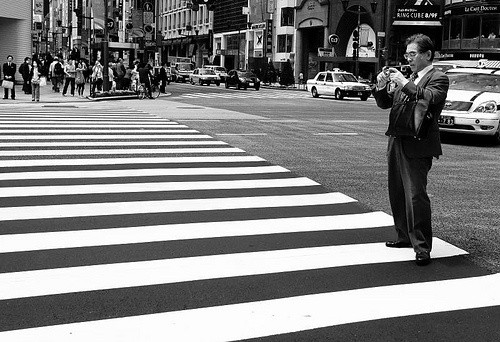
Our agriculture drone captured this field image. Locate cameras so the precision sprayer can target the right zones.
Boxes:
[383,69,394,81]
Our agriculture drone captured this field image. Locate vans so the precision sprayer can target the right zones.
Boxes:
[204,65,228,82]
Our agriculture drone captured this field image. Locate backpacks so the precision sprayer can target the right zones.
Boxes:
[54,61,62,75]
[19,64,25,74]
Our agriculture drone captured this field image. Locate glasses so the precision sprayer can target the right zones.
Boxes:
[403,53,416,59]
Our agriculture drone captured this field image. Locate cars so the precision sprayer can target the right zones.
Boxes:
[225,68,260,90]
[306,68,372,101]
[436,68,500,136]
[432,62,462,73]
[189,68,221,86]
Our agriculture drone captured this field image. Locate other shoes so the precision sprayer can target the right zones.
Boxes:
[386,240,413,248]
[416,251,430,266]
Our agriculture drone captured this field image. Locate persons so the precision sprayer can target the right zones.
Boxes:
[488,33,496,40]
[0,50,168,102]
[371,34,449,265]
[299,72,304,85]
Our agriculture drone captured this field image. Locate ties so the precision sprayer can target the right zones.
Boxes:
[398,73,418,103]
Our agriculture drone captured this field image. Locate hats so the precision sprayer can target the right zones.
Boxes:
[25,57,31,62]
[134,61,140,64]
[54,56,58,60]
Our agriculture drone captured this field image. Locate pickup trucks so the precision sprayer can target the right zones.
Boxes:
[170,62,195,83]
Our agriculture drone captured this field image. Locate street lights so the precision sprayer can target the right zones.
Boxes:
[341,0,378,78]
[227,20,252,69]
[57,20,81,50]
[177,29,200,62]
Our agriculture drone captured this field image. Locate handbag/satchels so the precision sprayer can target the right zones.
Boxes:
[39,77,47,86]
[384,85,430,141]
[112,81,116,88]
[22,83,32,92]
[2,80,14,89]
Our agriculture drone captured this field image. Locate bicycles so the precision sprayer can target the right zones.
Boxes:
[138,75,160,100]
